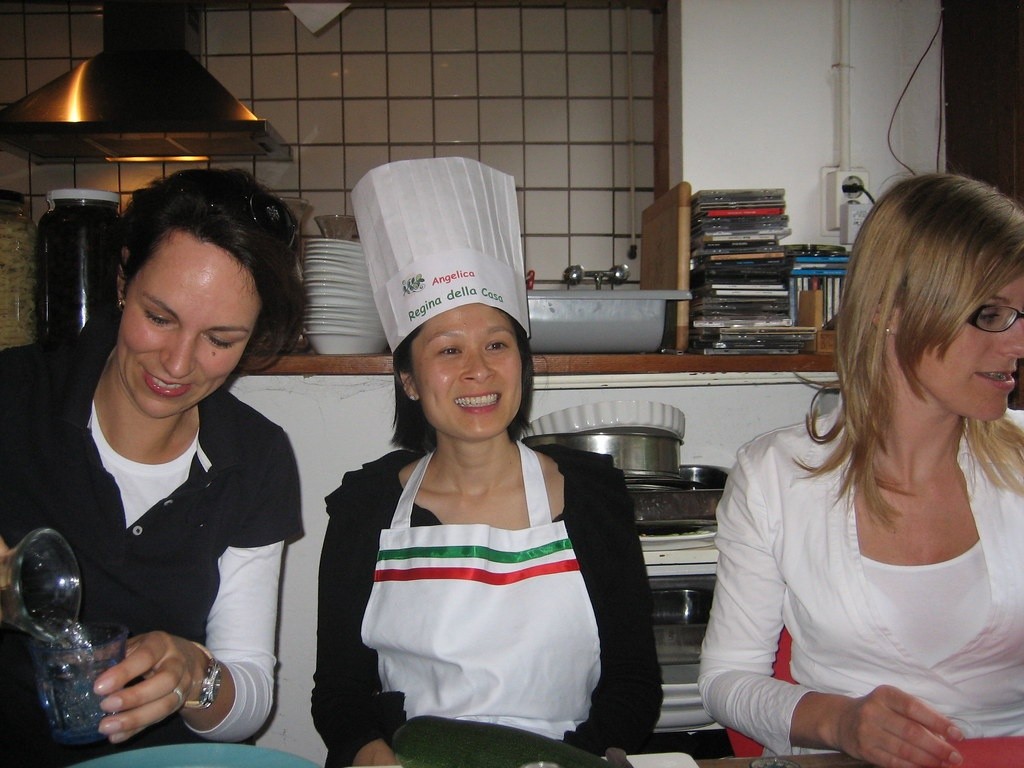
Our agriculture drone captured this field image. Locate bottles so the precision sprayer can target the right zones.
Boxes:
[37,189,122,340]
[0,188,42,348]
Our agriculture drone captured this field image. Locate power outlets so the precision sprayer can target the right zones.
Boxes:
[825,168,870,231]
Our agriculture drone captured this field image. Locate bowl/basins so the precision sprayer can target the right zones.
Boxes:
[303,238,388,354]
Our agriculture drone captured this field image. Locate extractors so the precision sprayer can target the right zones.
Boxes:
[0,0,294,161]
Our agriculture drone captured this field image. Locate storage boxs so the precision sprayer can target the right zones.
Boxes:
[528,290,693,354]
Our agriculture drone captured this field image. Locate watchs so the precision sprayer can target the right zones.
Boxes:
[183,641,222,710]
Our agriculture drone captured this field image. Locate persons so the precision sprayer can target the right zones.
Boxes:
[698,173,1024,768]
[0,167,301,768]
[310,303,665,768]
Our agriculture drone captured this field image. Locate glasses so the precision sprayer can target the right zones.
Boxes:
[160,170,296,250]
[965,304,1024,332]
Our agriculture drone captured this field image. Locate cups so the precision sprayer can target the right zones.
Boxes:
[25,621,129,744]
[0,528,82,641]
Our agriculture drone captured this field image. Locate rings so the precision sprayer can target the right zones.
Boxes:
[172,687,185,705]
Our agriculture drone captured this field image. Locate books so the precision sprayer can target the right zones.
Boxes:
[687,188,851,356]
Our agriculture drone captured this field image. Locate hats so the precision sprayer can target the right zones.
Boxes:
[351,156,531,354]
